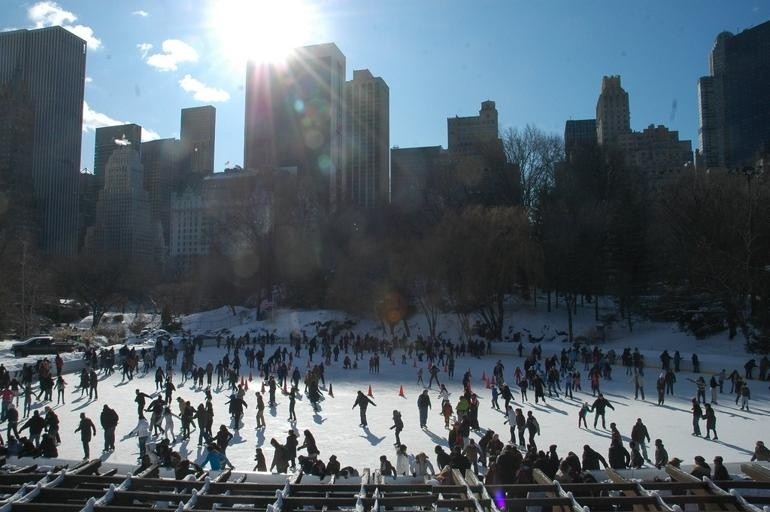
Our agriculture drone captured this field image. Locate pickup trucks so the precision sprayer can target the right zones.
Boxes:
[12,337,77,360]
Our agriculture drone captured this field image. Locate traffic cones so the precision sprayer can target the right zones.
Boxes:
[327,383,333,395]
[366,384,374,397]
[398,384,404,396]
[444,364,448,372]
[427,359,432,370]
[281,383,288,394]
[307,359,311,369]
[479,371,495,389]
[240,361,282,392]
[390,359,396,365]
[412,358,417,368]
